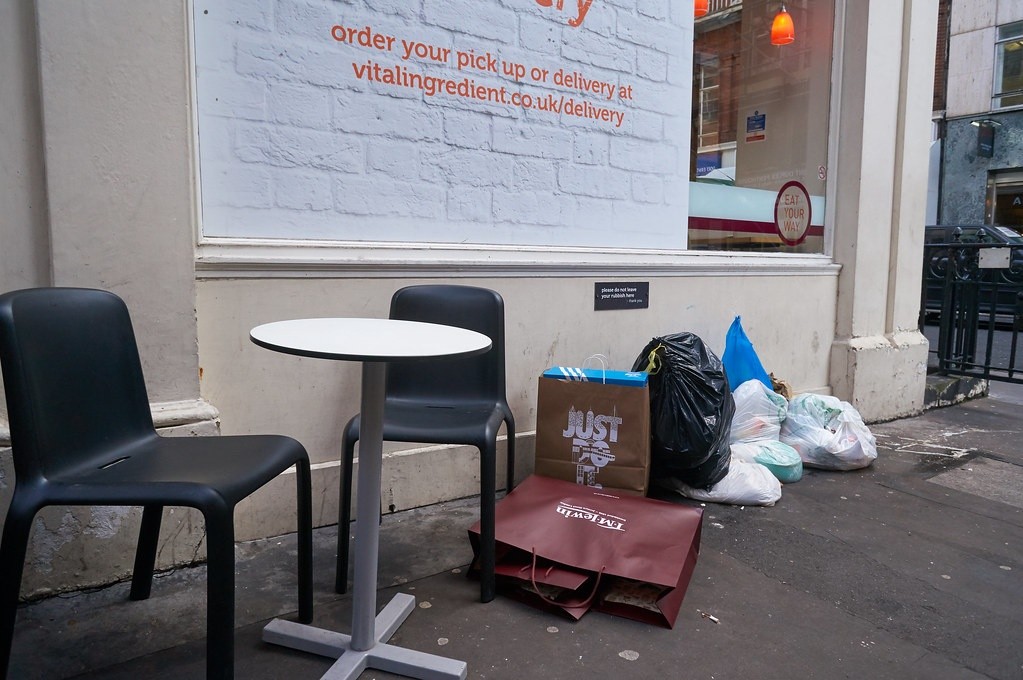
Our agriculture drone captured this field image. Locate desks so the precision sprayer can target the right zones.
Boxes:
[248,317,493,680]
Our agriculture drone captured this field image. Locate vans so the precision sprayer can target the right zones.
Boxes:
[924,224,1023,324]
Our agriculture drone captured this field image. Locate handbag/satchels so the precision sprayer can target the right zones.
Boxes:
[677,379,879,506]
[533,354,650,493]
[467,475,704,632]
[490,557,593,621]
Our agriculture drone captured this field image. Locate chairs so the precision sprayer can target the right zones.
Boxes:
[334,285,515,603]
[0,286,313,680]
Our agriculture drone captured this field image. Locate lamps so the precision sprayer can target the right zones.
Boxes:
[694,0,709,17]
[770,0,795,46]
